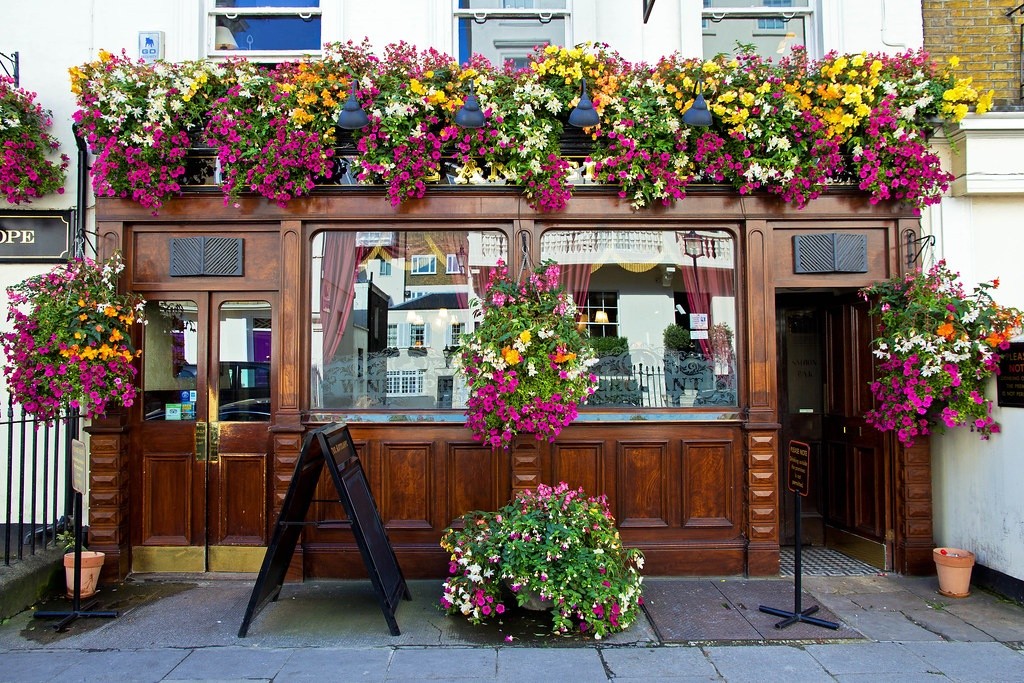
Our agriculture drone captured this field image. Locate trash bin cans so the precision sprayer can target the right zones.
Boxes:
[933,547,976,597]
[62,551,105,599]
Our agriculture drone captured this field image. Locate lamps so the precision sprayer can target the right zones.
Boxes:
[337,79,370,130]
[567,77,600,128]
[682,80,713,127]
[456,78,487,128]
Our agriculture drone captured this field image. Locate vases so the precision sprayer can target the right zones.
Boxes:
[917,401,945,426]
[65,552,105,598]
[517,586,557,612]
[933,547,975,598]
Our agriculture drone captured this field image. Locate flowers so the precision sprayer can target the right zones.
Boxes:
[857,259,1024,448]
[0,237,149,431]
[447,259,600,452]
[439,481,647,641]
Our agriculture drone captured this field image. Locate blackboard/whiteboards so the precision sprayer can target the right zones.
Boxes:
[242,422,414,620]
